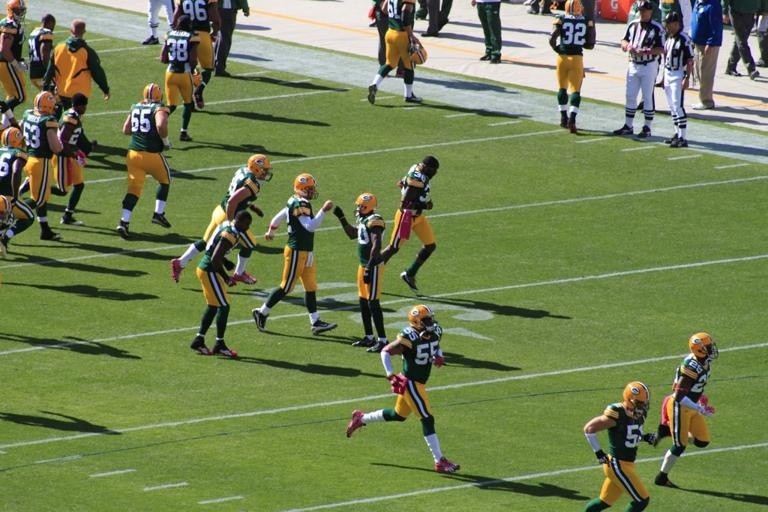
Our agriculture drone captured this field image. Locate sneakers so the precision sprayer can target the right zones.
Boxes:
[405,94,423,102]
[242,273,256,285]
[368,85,377,103]
[654,471,680,488]
[653,420,671,448]
[195,89,204,108]
[179,132,192,142]
[171,259,181,283]
[252,309,268,331]
[190,336,211,355]
[212,345,239,357]
[213,68,231,77]
[311,318,338,336]
[479,51,502,65]
[0,176,172,258]
[433,459,460,474]
[560,60,768,148]
[346,409,366,438]
[400,271,420,292]
[351,336,376,346]
[142,35,159,45]
[366,340,390,353]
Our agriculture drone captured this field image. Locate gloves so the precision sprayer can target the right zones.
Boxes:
[644,432,657,447]
[387,374,405,396]
[594,449,610,467]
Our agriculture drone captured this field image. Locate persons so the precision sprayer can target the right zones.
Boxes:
[0,127,36,258]
[117,82,177,238]
[252,170,338,337]
[142,1,252,146]
[524,1,556,16]
[334,189,388,353]
[344,302,460,477]
[650,331,722,491]
[610,0,767,149]
[358,1,455,107]
[372,153,441,289]
[169,153,273,285]
[20,13,111,242]
[2,1,29,124]
[547,0,596,134]
[469,0,505,63]
[188,210,253,361]
[576,381,656,512]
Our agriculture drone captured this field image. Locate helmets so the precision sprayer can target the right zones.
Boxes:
[622,381,650,418]
[33,91,56,114]
[1,126,25,149]
[688,333,719,362]
[355,192,376,215]
[408,305,437,331]
[564,0,585,16]
[143,81,162,103]
[8,0,27,21]
[247,154,273,183]
[408,43,428,64]
[294,173,319,200]
[700,406,715,417]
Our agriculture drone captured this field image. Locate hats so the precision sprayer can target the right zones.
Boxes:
[635,3,654,12]
[71,92,88,107]
[662,11,681,23]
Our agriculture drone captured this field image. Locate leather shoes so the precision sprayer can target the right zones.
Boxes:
[421,19,449,37]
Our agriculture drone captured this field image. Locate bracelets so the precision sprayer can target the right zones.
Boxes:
[269,225,279,231]
